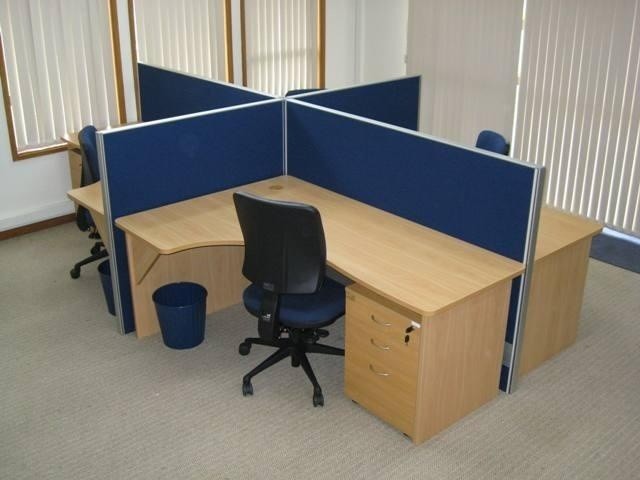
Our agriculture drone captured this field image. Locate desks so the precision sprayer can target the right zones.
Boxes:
[62,122,149,257]
[114,174,526,447]
[515,205,603,381]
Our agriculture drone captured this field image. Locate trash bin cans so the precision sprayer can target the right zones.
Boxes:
[98,259,115,316]
[152,281,208,349]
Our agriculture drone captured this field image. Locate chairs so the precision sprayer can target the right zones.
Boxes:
[285,89,320,95]
[233,193,346,406]
[476,130,509,157]
[71,125,108,278]
